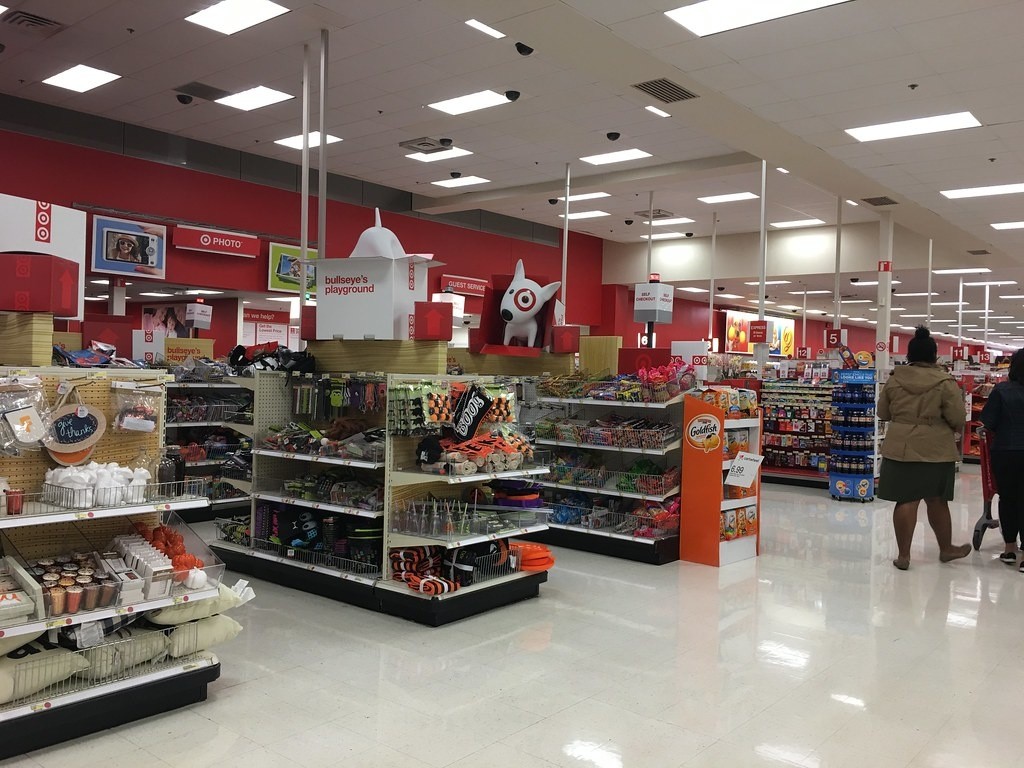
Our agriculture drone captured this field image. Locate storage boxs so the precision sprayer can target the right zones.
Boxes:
[479,273,566,357]
[305,253,447,340]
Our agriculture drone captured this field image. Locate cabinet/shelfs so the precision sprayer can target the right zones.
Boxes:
[0,360,1009,764]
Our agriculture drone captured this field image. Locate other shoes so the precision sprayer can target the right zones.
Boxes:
[893,556,910,570]
[939,544,971,561]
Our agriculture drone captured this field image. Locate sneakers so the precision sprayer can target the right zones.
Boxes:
[999,552,1017,563]
[1019,561,1024,571]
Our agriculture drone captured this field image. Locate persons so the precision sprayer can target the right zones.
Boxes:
[979,348,1024,573]
[144,306,190,338]
[111,234,140,262]
[876,326,972,569]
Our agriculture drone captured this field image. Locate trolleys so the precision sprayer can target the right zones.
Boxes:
[973,427,999,551]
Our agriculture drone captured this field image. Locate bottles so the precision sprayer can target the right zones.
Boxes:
[831,386,874,403]
[829,456,874,475]
[830,432,874,450]
[394,508,479,537]
[831,410,874,427]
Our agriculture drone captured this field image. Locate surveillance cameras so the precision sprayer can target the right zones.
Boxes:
[686,232,693,237]
[516,43,534,56]
[505,91,520,102]
[850,278,859,282]
[451,172,461,178]
[177,95,193,104]
[718,287,725,291]
[822,313,826,315]
[606,132,620,140]
[625,220,633,225]
[792,310,796,312]
[765,296,768,298]
[892,289,896,292]
[440,138,452,146]
[548,199,558,204]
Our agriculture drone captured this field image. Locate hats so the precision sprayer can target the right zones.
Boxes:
[905,324,937,363]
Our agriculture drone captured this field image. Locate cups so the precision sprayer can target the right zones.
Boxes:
[23,553,118,615]
[4,488,25,514]
